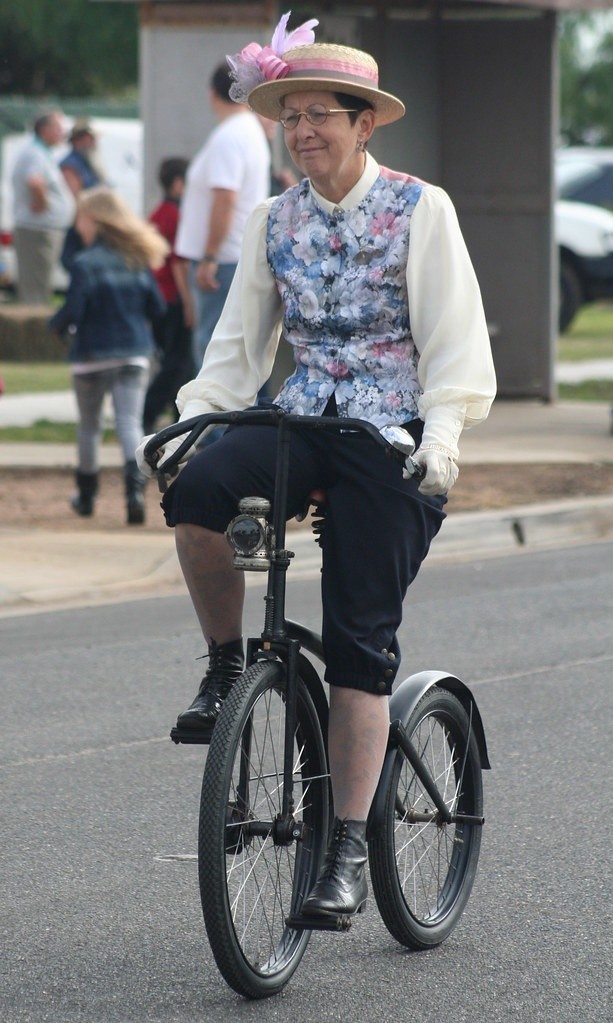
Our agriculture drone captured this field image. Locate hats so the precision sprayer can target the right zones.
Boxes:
[67,124,95,142]
[226,10,405,128]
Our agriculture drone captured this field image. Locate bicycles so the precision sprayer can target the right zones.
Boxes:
[136,406,495,1002]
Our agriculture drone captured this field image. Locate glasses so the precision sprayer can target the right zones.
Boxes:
[275,102,361,129]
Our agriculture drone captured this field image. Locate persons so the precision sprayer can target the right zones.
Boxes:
[176,61,297,407]
[12,115,66,299]
[134,11,497,914]
[50,189,170,523]
[60,123,104,273]
[142,158,193,437]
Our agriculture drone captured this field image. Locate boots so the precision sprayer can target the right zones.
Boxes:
[301,814,369,916]
[125,460,146,523]
[177,639,245,728]
[70,467,99,517]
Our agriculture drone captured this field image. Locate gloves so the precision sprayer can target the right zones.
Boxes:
[133,430,197,479]
[403,450,459,496]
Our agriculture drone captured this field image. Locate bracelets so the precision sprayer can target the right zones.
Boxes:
[201,255,217,263]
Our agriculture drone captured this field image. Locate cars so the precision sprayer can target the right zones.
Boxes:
[554,198,613,333]
[556,145,613,211]
[0,118,145,292]
